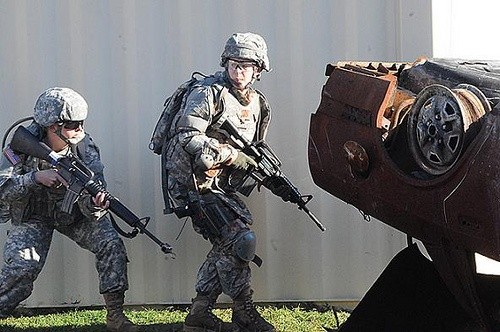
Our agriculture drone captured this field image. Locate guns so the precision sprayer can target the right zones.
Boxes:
[10,125,177,260]
[219,118,326,232]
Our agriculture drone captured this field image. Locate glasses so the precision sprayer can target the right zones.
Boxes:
[63,120,84,129]
[225,62,256,73]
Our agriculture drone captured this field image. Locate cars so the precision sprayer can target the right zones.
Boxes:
[307,57,499,332]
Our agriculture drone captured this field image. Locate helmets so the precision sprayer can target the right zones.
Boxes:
[220,32,273,81]
[32,86,89,127]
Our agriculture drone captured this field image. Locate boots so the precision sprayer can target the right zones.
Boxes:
[184,292,233,332]
[103,292,143,332]
[232,288,276,332]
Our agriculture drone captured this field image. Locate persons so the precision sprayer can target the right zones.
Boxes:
[153,32,290,332]
[0,87,137,332]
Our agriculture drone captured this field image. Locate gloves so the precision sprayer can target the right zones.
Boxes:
[230,151,258,171]
